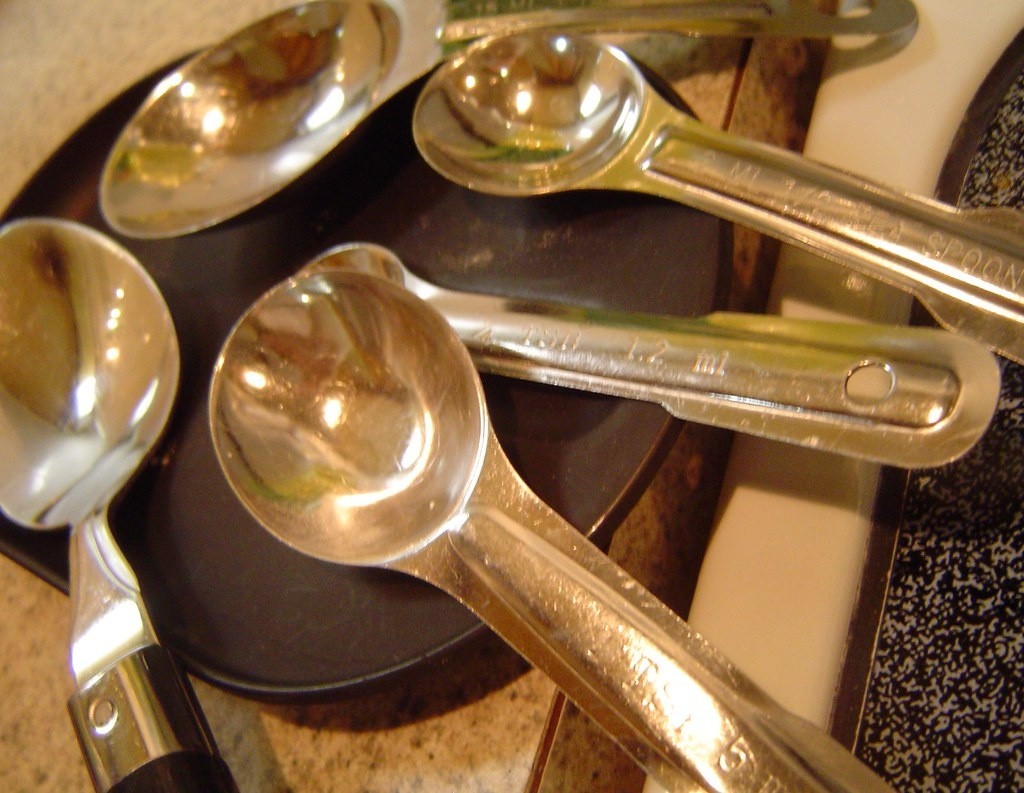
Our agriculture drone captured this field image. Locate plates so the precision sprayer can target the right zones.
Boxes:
[0,46,737,703]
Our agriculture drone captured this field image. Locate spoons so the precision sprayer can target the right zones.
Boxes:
[97,0,902,241]
[413,27,1024,368]
[207,270,896,793]
[298,242,1002,473]
[0,216,240,793]
